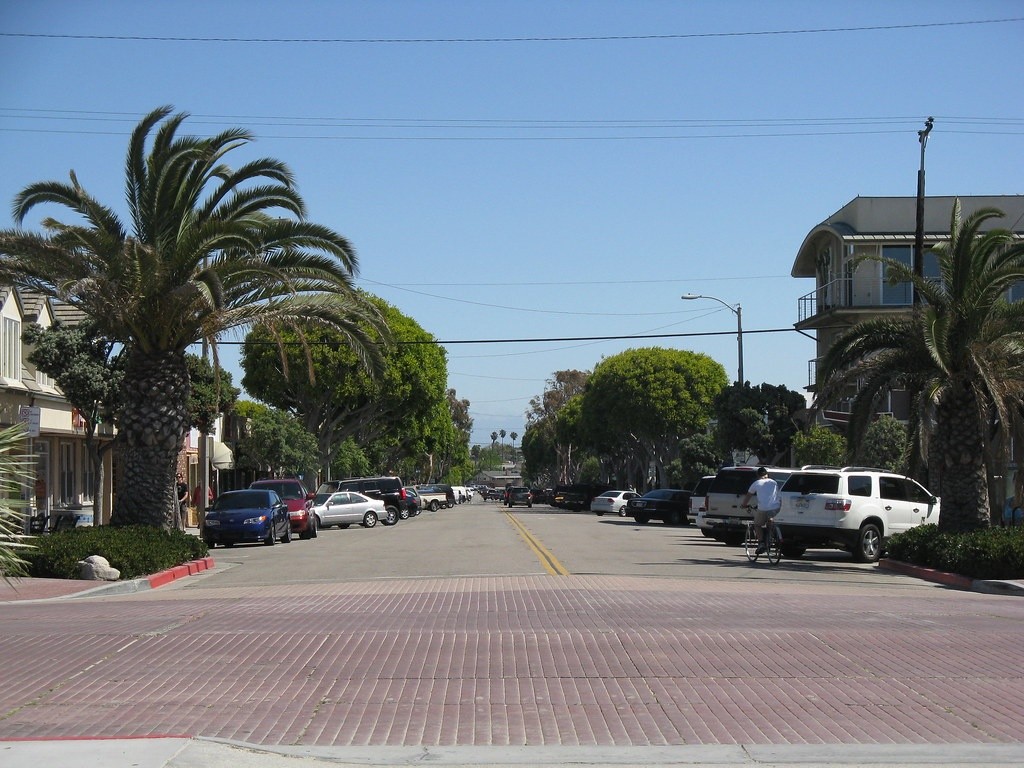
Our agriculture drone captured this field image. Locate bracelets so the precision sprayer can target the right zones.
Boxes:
[742,505,744,507]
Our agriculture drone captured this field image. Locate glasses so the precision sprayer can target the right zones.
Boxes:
[176,475,181,478]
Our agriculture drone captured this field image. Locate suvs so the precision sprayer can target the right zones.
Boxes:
[770,464,941,564]
[248,477,318,540]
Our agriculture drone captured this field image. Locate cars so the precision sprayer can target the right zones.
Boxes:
[687,477,716,538]
[312,492,388,529]
[626,490,695,527]
[398,482,475,520]
[548,481,613,512]
[590,490,641,518]
[508,487,533,508]
[473,484,515,506]
[703,467,801,547]
[204,490,293,549]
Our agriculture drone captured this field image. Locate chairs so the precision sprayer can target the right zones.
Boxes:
[30,513,51,536]
[44,515,64,533]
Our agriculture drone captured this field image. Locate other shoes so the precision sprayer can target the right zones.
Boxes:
[755,543,766,555]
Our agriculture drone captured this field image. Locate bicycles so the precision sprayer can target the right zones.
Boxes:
[741,504,783,565]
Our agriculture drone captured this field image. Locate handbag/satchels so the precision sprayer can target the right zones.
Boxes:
[208,497,215,505]
[181,503,188,512]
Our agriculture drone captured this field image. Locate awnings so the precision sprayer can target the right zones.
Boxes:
[210,441,234,471]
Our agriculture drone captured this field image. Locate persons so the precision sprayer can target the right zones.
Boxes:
[1004,470,1024,522]
[191,479,212,529]
[176,472,188,533]
[741,467,782,553]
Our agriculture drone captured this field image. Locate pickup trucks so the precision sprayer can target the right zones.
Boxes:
[402,486,448,512]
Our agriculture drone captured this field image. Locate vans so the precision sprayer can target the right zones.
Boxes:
[315,476,407,527]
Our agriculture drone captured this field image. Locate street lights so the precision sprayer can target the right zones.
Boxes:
[681,294,743,385]
